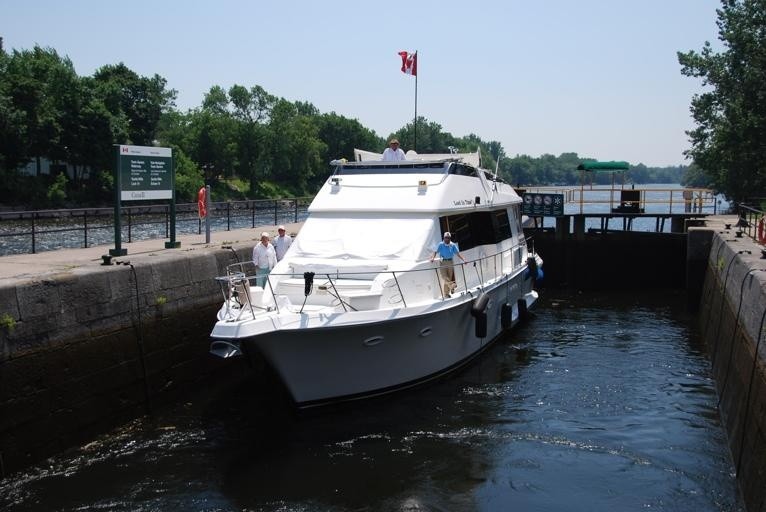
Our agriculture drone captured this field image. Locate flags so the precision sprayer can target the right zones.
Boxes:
[397,51,416,78]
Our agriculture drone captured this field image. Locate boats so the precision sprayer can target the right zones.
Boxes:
[209,145,544,415]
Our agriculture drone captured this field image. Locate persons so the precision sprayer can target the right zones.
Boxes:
[431,232,469,298]
[273,224,292,263]
[250,231,277,288]
[380,138,407,161]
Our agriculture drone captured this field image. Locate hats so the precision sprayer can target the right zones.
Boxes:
[443,231,450,238]
[277,225,284,231]
[260,231,268,238]
[389,138,399,146]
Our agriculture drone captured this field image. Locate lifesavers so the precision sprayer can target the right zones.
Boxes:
[198,188,207,217]
[758,219,765,245]
